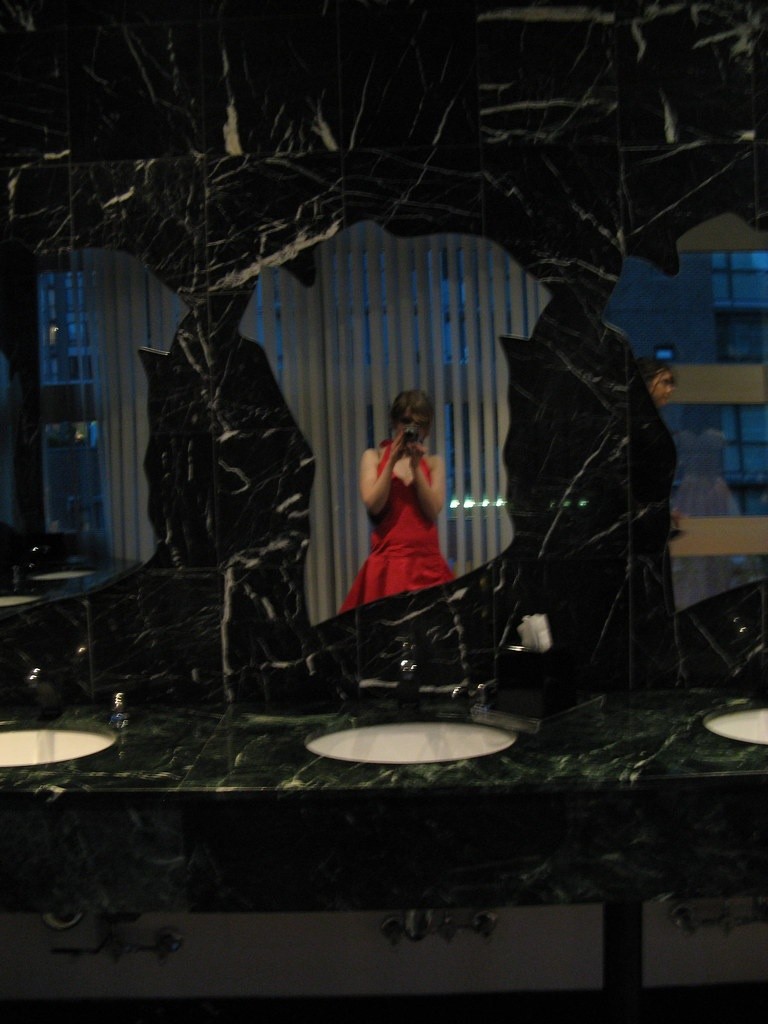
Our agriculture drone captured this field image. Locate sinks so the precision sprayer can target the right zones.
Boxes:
[700,696,768,744]
[29,567,99,582]
[302,716,522,766]
[0,594,40,610]
[0,722,117,771]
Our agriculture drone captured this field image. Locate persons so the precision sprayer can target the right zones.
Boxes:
[639,355,730,539]
[337,389,456,615]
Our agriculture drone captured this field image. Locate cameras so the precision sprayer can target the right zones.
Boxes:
[402,425,421,452]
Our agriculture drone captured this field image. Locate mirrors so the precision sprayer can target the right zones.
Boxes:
[197,0,634,704]
[0,0,197,711]
[615,0,768,695]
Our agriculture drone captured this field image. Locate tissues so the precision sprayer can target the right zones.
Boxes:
[494,613,584,715]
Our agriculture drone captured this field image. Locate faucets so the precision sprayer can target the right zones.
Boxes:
[6,545,41,585]
[396,657,422,707]
